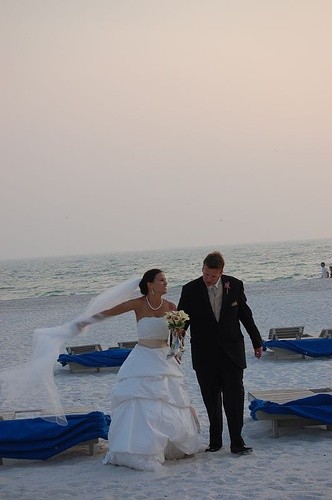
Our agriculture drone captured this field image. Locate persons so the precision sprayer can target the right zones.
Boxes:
[170,252,263,454]
[70,269,201,470]
[321,262,332,278]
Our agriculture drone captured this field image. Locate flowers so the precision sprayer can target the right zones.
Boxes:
[225,282,230,294]
[163,310,190,359]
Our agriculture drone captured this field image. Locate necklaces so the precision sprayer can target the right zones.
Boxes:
[145,296,163,310]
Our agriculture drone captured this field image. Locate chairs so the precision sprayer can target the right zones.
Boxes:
[0,326,332,466]
